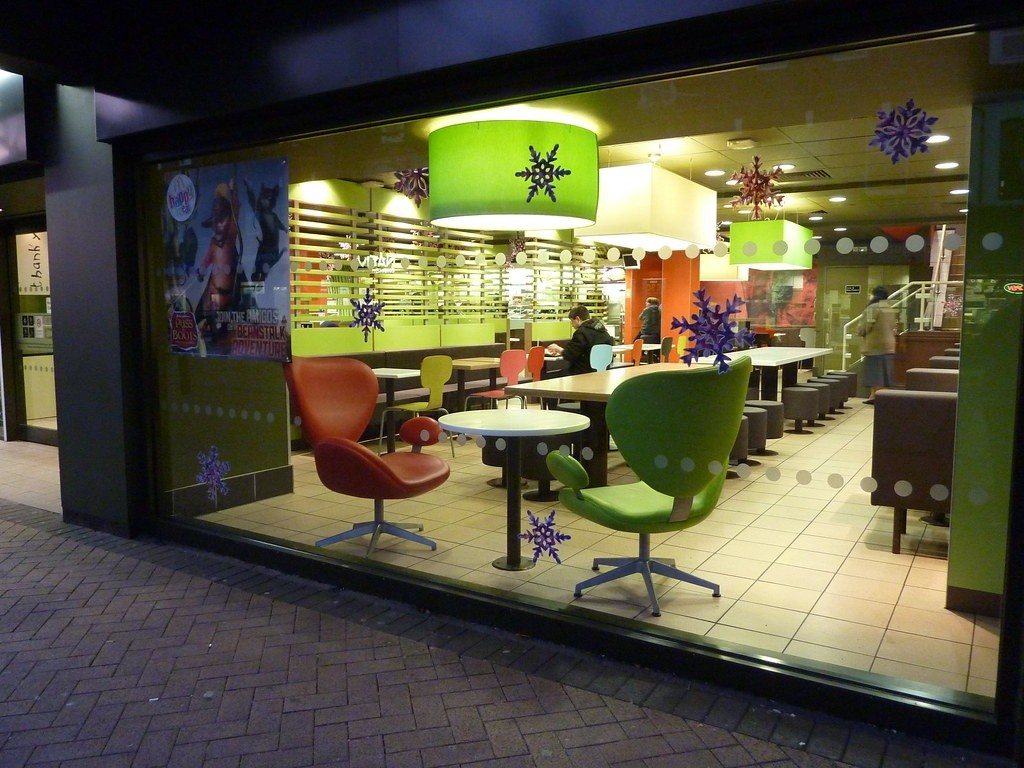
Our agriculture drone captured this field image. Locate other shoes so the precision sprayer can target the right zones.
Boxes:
[862,399,875,405]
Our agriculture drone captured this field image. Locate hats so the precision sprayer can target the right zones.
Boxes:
[873,286,888,300]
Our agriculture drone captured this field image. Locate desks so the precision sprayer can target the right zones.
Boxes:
[613,343,661,364]
[450,357,500,412]
[504,362,709,491]
[370,368,421,457]
[524,354,564,362]
[438,409,591,570]
[691,347,834,402]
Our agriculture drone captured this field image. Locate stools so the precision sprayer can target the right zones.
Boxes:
[818,375,853,410]
[728,415,761,467]
[807,378,844,415]
[743,406,767,449]
[745,400,784,456]
[796,383,835,427]
[827,371,857,409]
[782,386,825,434]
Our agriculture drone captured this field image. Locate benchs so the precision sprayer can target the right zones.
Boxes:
[355,339,570,442]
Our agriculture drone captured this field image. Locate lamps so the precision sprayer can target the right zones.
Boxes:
[729,198,814,270]
[574,144,717,252]
[420,104,600,231]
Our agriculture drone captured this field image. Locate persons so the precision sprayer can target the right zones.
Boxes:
[857,287,898,405]
[320,321,341,327]
[633,297,661,362]
[540,307,614,409]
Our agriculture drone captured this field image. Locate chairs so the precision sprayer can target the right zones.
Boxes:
[283,355,451,558]
[545,355,754,617]
[661,337,673,363]
[871,343,960,554]
[464,349,524,411]
[631,339,643,366]
[378,355,455,458]
[677,336,687,363]
[590,344,613,371]
[495,346,545,410]
